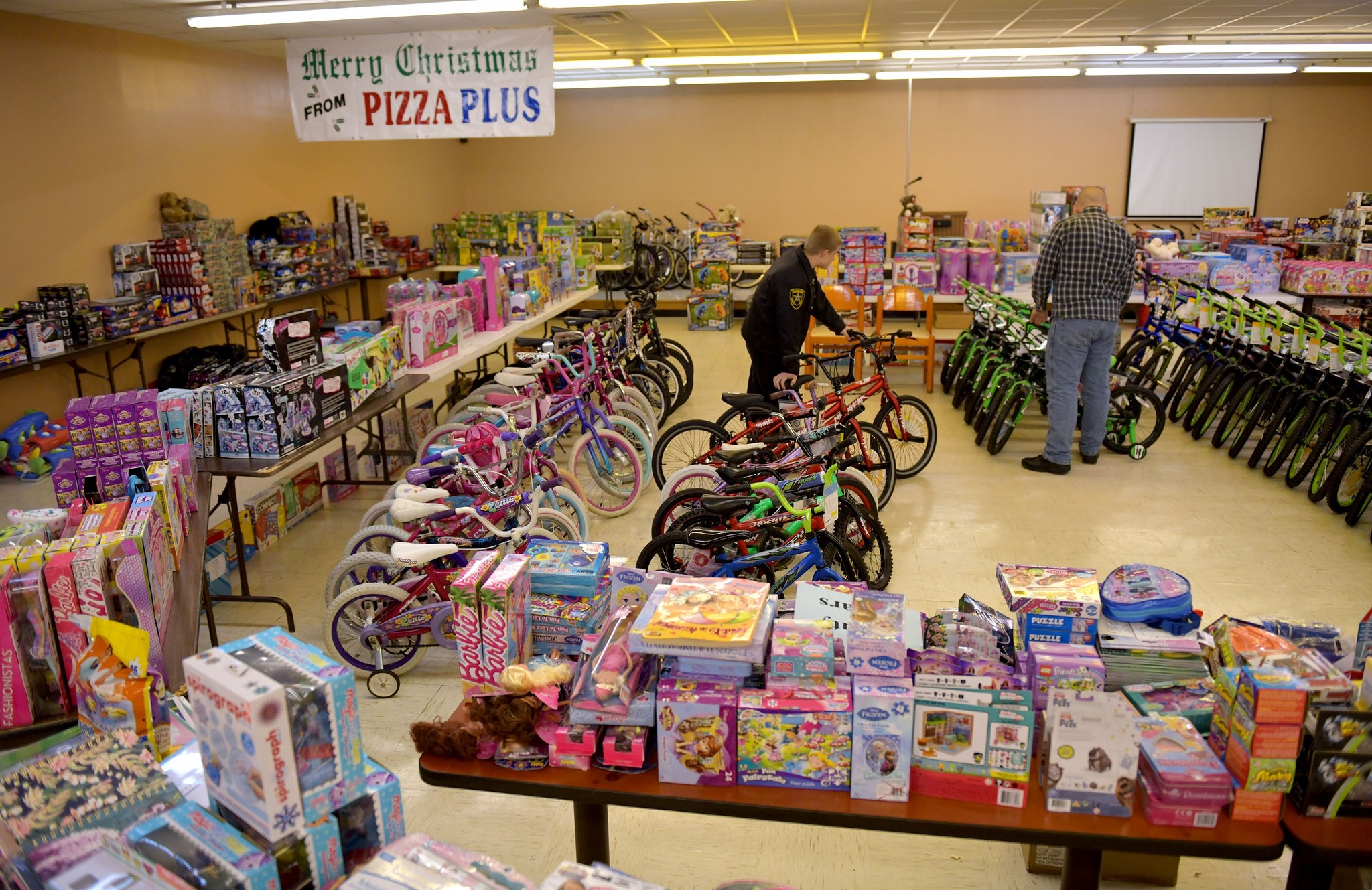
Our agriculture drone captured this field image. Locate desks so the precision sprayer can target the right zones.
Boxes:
[352,262,443,318]
[0,339,129,408]
[266,281,365,335]
[437,258,637,320]
[139,469,222,690]
[353,285,604,465]
[1276,782,1372,890]
[419,648,1286,890]
[195,372,432,633]
[128,300,265,381]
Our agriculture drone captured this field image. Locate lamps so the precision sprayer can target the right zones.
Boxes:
[185,0,1372,91]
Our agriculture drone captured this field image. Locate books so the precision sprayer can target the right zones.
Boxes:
[628,577,778,662]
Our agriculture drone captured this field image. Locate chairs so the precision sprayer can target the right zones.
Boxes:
[873,284,936,395]
[802,285,865,388]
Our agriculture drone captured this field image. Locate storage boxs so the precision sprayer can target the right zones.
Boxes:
[2,193,636,467]
[447,534,1372,836]
[687,191,1372,337]
[1,469,404,889]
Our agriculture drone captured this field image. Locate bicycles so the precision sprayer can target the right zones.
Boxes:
[634,329,938,601]
[319,283,697,699]
[595,202,771,291]
[941,268,1372,545]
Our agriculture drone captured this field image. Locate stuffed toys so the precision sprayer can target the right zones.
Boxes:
[1147,238,1179,260]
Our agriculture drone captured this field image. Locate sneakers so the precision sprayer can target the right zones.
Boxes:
[1080,451,1099,464]
[1022,455,1071,475]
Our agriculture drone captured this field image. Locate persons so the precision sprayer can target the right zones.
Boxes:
[604,247,619,264]
[23,576,63,705]
[741,224,851,449]
[494,648,574,693]
[1309,219,1322,234]
[1135,252,1145,287]
[1267,251,1281,274]
[361,334,403,386]
[1021,185,1137,477]
[593,621,641,702]
